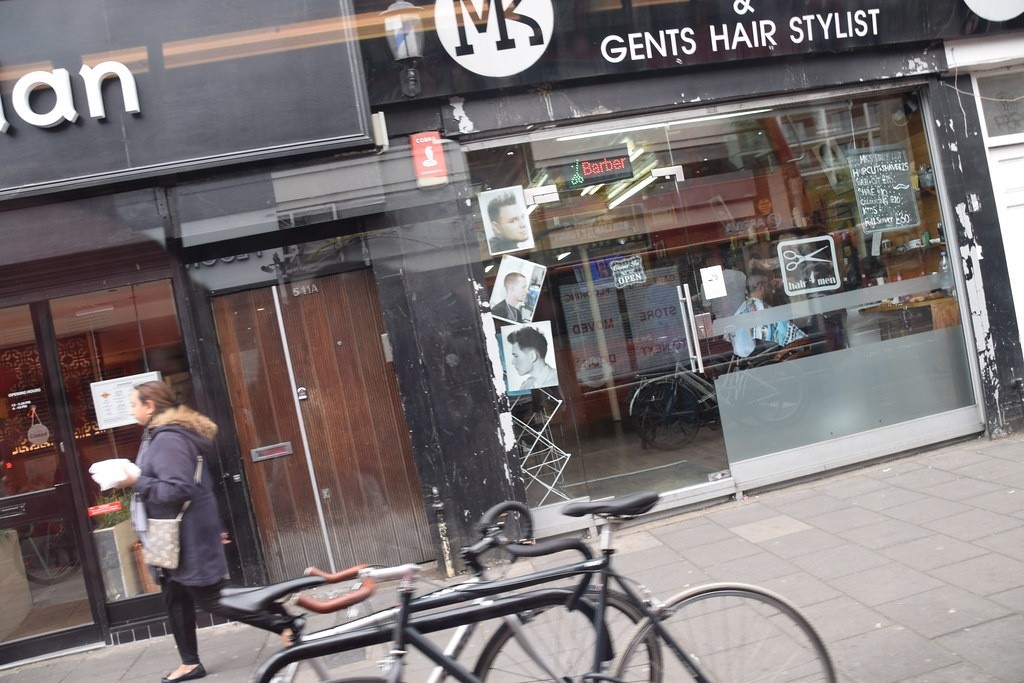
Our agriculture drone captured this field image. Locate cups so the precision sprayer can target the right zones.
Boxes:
[918,171,934,187]
[876,277,884,285]
[906,239,922,247]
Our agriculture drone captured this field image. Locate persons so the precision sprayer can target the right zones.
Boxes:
[727,272,811,355]
[507,327,559,390]
[113,382,299,683]
[493,272,528,323]
[819,144,853,195]
[488,191,529,254]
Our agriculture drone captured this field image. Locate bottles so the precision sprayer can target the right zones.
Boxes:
[132,492,148,532]
[939,251,953,296]
[843,277,850,291]
[861,273,866,288]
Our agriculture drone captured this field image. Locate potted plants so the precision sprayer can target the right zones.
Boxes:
[90,488,141,602]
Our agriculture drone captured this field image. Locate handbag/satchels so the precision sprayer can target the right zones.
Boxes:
[138,518,181,569]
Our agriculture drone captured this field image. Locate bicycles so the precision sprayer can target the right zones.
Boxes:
[320,489,843,683]
[11,490,117,586]
[217,490,666,683]
[629,332,810,453]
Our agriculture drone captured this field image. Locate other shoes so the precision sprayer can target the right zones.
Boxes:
[162,663,206,683]
[284,617,305,646]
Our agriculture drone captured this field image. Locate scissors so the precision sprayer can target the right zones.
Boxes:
[783,245,831,271]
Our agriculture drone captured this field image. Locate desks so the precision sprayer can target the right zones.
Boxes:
[866,297,961,342]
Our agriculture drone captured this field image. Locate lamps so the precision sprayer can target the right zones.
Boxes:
[380,0,425,97]
